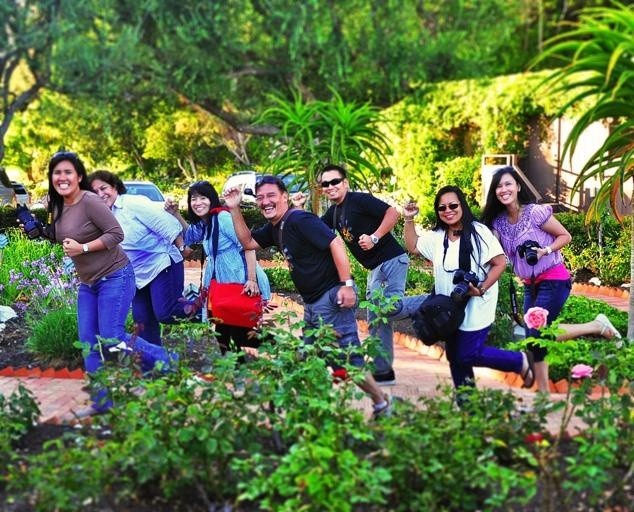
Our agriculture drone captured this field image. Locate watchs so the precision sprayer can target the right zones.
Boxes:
[340,279,354,287]
[370,233,378,244]
[179,244,186,252]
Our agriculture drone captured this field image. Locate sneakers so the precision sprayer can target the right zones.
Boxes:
[370,394,392,423]
[372,369,396,385]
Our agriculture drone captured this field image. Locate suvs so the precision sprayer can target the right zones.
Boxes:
[10,181,32,211]
[220,170,308,205]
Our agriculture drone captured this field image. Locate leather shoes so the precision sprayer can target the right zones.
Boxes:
[595,312,621,342]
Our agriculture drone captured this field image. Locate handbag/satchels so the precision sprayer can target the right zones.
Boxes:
[411,294,465,347]
[208,280,263,328]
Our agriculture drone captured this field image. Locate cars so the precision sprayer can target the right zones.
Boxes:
[121,181,166,211]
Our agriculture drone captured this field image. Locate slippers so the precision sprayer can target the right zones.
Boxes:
[521,350,536,388]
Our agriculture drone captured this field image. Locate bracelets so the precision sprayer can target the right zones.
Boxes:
[83,244,89,253]
[403,218,415,225]
[544,246,552,256]
[480,286,486,296]
[248,277,258,281]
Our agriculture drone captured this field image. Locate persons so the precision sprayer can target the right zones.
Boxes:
[14,151,181,418]
[289,166,434,386]
[480,166,622,412]
[163,180,271,397]
[222,175,394,418]
[87,170,206,380]
[402,187,537,407]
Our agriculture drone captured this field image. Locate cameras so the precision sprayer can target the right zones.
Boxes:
[517,240,543,266]
[451,270,479,304]
[18,210,45,240]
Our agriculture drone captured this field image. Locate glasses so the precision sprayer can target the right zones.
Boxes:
[189,181,209,188]
[437,203,459,211]
[55,151,77,159]
[255,177,278,184]
[321,178,342,188]
